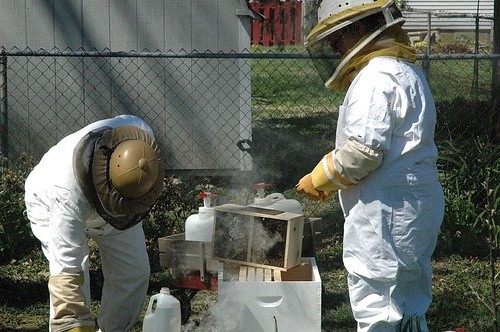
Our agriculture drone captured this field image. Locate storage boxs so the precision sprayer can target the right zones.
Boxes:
[158,233,217,289]
[211,257,321,332]
[213,203,304,272]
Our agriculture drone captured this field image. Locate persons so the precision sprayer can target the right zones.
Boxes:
[24,114,164,332]
[294,0,443,332]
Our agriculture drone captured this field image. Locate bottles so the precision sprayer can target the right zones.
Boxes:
[142,287,181,332]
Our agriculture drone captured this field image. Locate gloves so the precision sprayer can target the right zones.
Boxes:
[294,145,356,203]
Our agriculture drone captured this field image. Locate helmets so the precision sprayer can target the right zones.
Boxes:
[72,124,165,231]
[303,0,407,89]
